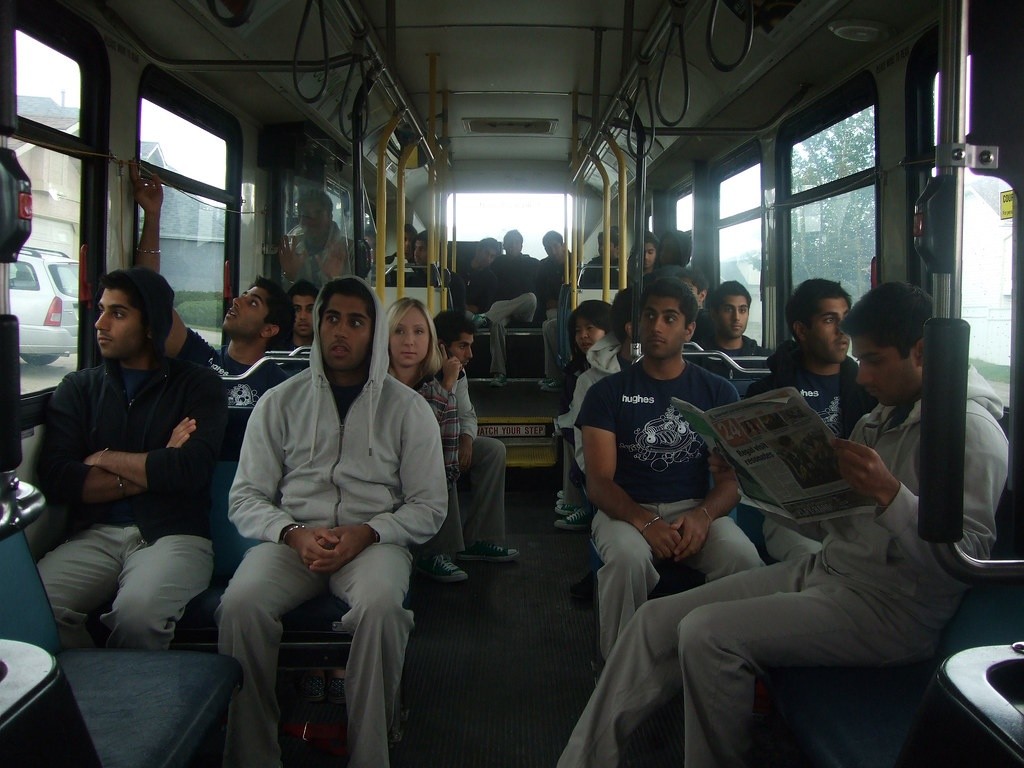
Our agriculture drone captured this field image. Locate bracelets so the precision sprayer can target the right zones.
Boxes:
[700,506,711,520]
[374,529,380,543]
[283,525,305,545]
[96,447,109,465]
[641,517,663,533]
[117,474,126,499]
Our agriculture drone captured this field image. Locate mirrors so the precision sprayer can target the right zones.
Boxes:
[386,121,428,169]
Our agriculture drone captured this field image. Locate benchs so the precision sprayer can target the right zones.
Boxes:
[0,242,1024,768]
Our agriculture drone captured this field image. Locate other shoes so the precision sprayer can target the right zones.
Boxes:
[570,571,594,600]
[327,668,345,704]
[555,490,564,505]
[300,672,325,703]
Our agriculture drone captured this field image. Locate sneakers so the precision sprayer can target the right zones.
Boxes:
[554,509,591,531]
[490,373,507,386]
[541,381,566,393]
[555,504,577,515]
[539,377,557,386]
[470,313,491,327]
[457,539,520,562]
[416,556,468,581]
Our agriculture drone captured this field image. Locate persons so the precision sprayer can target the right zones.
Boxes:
[746,420,762,435]
[125,155,291,462]
[777,434,819,490]
[533,236,581,307]
[538,223,774,529]
[450,235,498,315]
[472,235,540,387]
[573,278,775,717]
[266,279,324,355]
[353,223,464,311]
[735,278,882,566]
[296,299,462,703]
[412,308,520,585]
[276,189,356,280]
[553,278,1009,768]
[217,274,447,768]
[27,266,228,655]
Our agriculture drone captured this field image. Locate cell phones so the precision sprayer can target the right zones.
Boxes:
[438,341,465,381]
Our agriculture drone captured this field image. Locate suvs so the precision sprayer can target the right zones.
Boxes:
[8,245,79,365]
